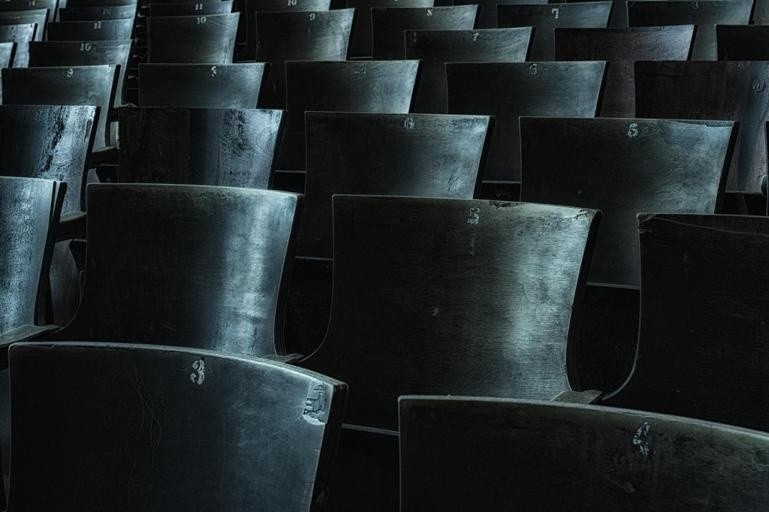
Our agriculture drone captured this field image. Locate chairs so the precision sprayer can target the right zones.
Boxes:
[1,0,768,511]
